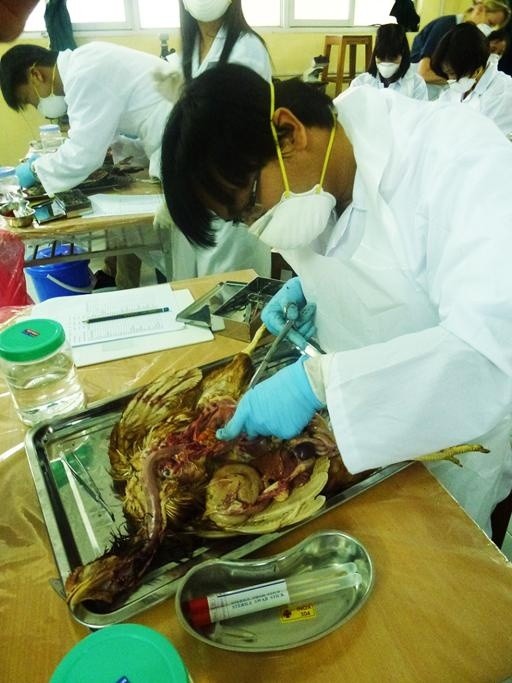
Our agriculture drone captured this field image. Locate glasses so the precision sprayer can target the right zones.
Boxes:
[231,170,262,225]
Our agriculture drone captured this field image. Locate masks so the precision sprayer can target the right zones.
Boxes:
[476,23,493,37]
[375,62,400,79]
[183,0,234,22]
[31,80,67,120]
[247,129,337,250]
[446,77,476,94]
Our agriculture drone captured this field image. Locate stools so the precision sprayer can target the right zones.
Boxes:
[322,35,371,99]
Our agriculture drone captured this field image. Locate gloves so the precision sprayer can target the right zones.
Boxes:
[15,154,38,188]
[261,277,316,350]
[215,353,325,441]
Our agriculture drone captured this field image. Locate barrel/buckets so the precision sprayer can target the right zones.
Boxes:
[22,243,99,299]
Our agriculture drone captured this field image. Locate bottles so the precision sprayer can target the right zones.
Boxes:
[0,317,86,428]
[184,561,363,627]
[0,165,20,193]
[38,124,62,153]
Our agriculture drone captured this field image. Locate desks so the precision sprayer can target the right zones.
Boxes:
[0,269,512,683]
[0,167,173,283]
